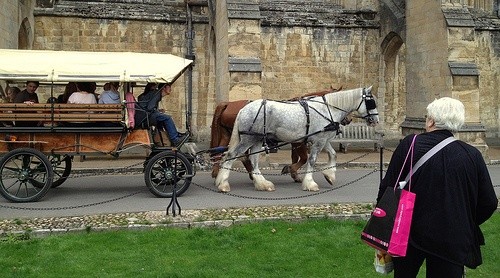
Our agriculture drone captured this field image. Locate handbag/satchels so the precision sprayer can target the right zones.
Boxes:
[373,249,393,275]
[360,187,417,258]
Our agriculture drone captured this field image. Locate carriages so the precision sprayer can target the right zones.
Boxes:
[0,47,380,203]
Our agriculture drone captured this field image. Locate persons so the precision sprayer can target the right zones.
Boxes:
[375,97,498,278]
[0,81,189,150]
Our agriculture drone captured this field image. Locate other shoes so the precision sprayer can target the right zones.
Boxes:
[174,131,190,150]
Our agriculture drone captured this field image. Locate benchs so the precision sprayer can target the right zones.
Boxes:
[0,101,130,134]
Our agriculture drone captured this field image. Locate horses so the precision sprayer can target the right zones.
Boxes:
[213,85,354,182]
[215,84,380,192]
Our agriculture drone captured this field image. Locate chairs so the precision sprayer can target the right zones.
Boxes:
[124,92,153,145]
